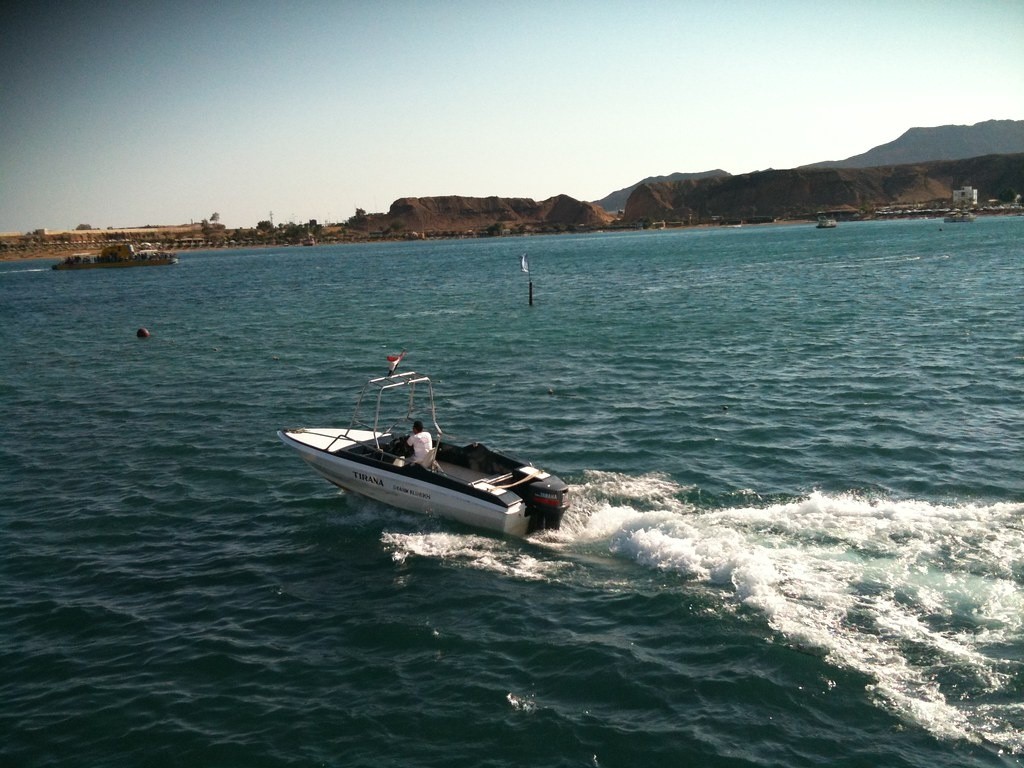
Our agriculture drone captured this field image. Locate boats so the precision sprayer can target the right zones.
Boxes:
[277,373,571,539]
[301,239,315,246]
[943,211,975,223]
[815,216,837,228]
[52,243,178,269]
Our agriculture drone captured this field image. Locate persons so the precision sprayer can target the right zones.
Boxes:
[404,421,432,462]
[67,252,176,265]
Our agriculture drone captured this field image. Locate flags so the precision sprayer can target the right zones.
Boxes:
[387,351,406,377]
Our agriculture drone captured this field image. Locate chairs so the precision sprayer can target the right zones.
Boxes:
[392,447,437,469]
